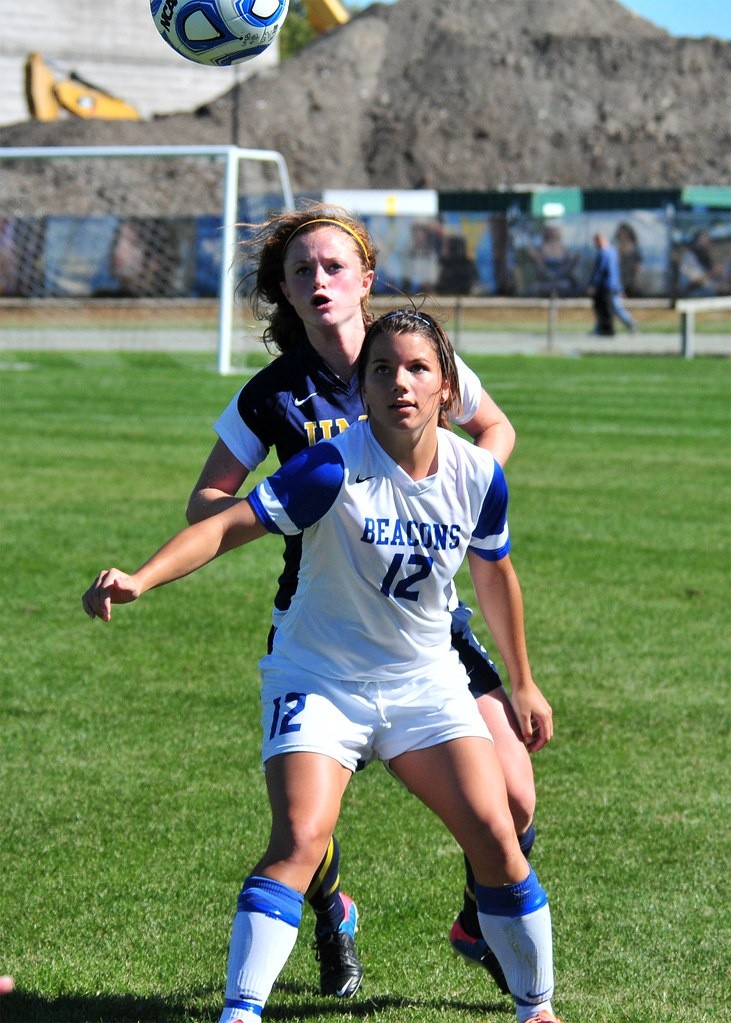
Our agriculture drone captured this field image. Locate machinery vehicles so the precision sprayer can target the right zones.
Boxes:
[26,52,140,124]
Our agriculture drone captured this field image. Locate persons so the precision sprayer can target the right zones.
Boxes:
[380,219,731,299]
[182,202,553,997]
[586,233,638,336]
[81,287,564,1023]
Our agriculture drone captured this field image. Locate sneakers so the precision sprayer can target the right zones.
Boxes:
[312,891,364,998]
[449,913,513,998]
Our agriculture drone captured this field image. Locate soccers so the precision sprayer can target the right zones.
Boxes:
[148,1,292,67]
